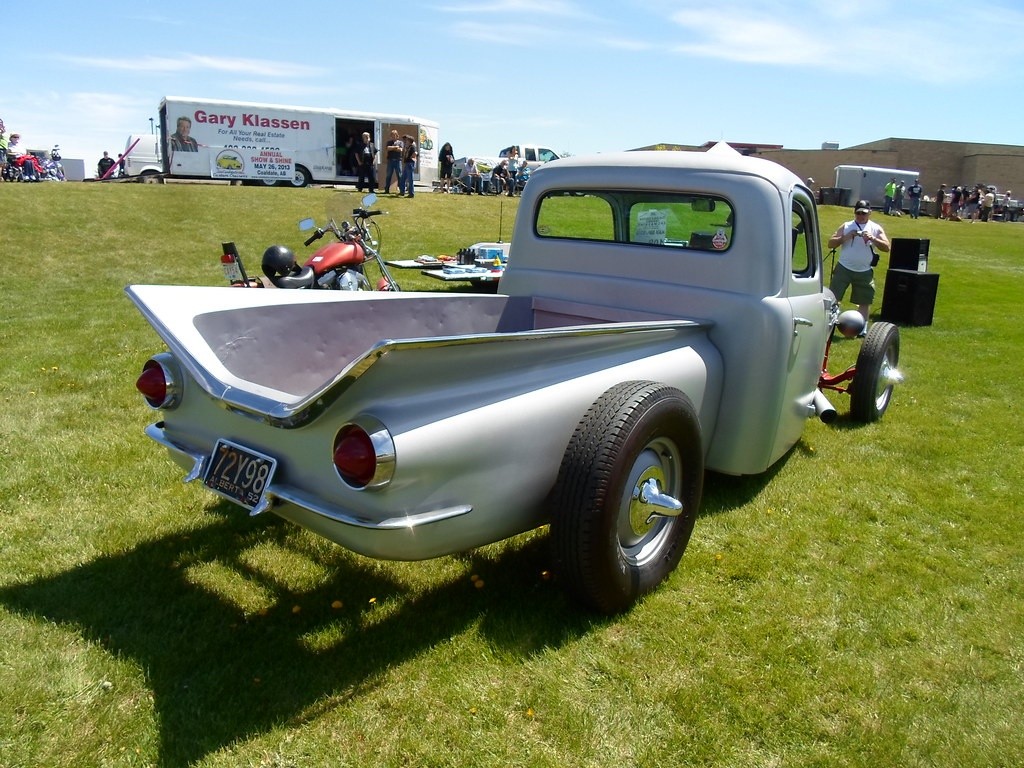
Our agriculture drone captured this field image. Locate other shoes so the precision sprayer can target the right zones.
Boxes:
[857,321,867,338]
[478,192,483,195]
[507,193,513,196]
[404,195,414,198]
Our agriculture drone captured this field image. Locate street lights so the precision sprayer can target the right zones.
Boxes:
[149,117,154,134]
[155,125,160,134]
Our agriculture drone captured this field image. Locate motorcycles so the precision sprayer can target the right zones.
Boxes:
[220,192,400,292]
[38,144,68,182]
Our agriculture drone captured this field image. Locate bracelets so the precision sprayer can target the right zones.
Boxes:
[871,236,876,242]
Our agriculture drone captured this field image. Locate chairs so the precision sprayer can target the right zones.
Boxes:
[690,232,714,249]
[454,164,524,196]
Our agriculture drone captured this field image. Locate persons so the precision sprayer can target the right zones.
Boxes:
[0,119,38,181]
[383,129,403,194]
[438,142,455,194]
[507,146,518,180]
[171,116,199,152]
[458,158,484,195]
[827,200,890,337]
[343,135,359,176]
[514,161,530,185]
[491,159,517,197]
[98,151,117,178]
[354,132,378,193]
[398,135,418,198]
[883,177,896,216]
[948,183,1012,222]
[118,153,126,176]
[935,184,947,219]
[895,180,906,209]
[907,178,923,220]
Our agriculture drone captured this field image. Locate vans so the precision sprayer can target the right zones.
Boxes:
[124,135,163,182]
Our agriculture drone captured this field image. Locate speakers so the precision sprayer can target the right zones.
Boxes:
[880,268,940,327]
[888,237,930,273]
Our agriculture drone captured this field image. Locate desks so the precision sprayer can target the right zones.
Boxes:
[385,259,507,281]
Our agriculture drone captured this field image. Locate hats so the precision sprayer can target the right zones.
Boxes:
[855,200,871,214]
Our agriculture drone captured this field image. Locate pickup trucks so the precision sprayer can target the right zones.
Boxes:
[124,150,903,619]
[451,145,585,197]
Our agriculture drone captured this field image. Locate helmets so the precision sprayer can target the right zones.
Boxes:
[262,245,302,279]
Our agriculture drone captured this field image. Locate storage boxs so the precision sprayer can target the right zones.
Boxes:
[478,248,503,262]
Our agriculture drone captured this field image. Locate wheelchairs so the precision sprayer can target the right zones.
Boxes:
[2,155,39,183]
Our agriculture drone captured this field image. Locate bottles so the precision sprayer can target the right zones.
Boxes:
[456,247,488,264]
[917,253,927,274]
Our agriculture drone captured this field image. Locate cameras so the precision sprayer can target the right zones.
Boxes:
[856,231,863,237]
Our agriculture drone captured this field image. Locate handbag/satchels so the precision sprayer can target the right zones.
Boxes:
[870,254,879,267]
